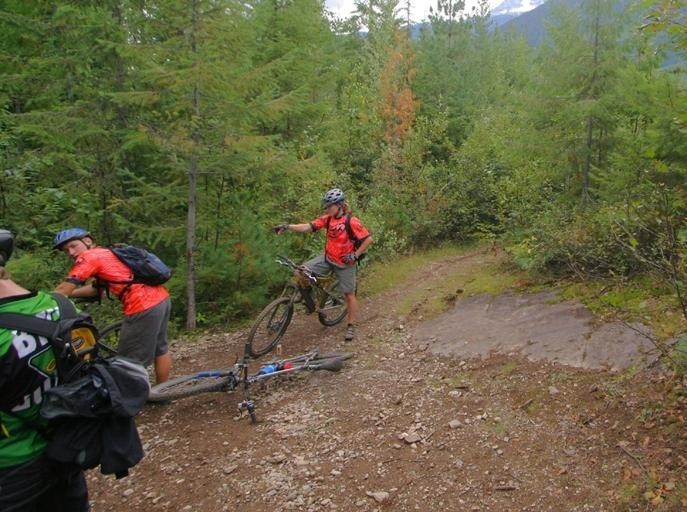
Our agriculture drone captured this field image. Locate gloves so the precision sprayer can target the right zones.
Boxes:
[270,224,288,235]
[340,253,358,264]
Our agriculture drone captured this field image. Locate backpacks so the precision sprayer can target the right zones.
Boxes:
[0,293,150,480]
[327,215,366,265]
[95,243,172,285]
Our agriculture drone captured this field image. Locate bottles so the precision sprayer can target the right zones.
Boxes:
[258,363,278,374]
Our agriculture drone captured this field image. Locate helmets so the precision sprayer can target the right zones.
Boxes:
[324,189,344,208]
[51,226,90,250]
[0,229,13,266]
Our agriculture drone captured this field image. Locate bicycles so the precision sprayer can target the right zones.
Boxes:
[244,254,359,359]
[146,349,356,427]
[67,294,123,360]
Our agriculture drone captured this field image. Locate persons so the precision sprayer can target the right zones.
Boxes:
[53,228,171,384]
[0,228,96,512]
[271,187,373,341]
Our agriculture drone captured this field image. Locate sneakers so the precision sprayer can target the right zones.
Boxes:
[345,326,353,340]
